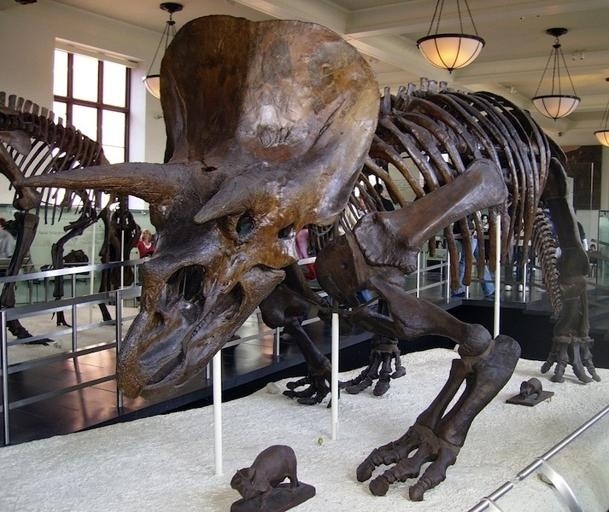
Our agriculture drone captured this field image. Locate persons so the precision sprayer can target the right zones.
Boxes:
[447,216,495,299]
[588,243,598,262]
[371,181,397,213]
[135,230,155,258]
[478,214,490,236]
[503,215,535,294]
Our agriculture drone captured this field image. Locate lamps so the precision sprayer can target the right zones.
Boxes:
[531,27,582,123]
[142,2,181,100]
[594,76,609,147]
[416,0,486,76]
